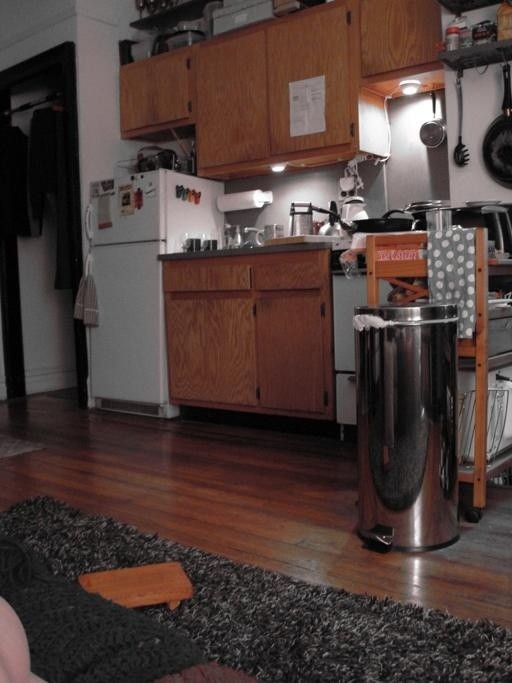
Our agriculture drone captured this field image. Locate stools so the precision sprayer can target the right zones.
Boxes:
[78,562,193,610]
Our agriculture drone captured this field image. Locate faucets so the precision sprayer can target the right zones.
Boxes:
[242,224,265,238]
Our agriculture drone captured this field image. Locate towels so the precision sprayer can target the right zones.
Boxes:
[426,224,476,339]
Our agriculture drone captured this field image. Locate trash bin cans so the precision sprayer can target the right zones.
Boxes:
[354,302,462,554]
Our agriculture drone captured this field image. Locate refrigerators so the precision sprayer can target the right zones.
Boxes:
[83,166,228,422]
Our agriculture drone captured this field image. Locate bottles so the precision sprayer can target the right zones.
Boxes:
[446,26,460,52]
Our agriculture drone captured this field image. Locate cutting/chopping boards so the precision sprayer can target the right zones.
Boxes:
[272,235,334,245]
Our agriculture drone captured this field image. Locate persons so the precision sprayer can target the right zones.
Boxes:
[1,596,261,683]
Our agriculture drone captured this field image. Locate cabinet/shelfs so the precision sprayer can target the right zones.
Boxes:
[367,227,512,524]
[163,250,335,421]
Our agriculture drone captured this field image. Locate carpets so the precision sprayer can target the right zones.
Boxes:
[0,495,512,683]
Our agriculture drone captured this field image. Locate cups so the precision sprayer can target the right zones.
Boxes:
[255,224,284,245]
[183,230,202,252]
[199,231,218,251]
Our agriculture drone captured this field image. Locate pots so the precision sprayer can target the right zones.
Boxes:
[420,92,447,150]
[351,206,415,233]
[481,61,512,191]
